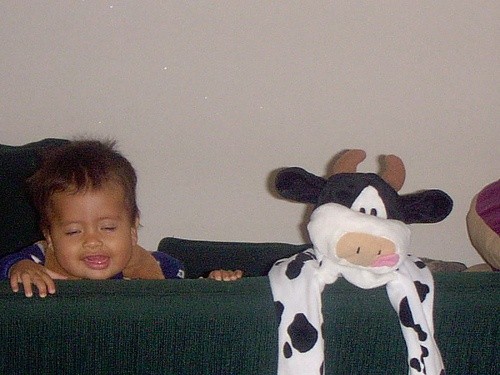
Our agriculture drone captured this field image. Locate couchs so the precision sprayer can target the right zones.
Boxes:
[0,137,499,374]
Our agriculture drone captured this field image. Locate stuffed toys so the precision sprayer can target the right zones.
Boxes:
[264,146,453,375]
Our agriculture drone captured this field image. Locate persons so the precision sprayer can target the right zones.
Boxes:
[0,138,243,300]
